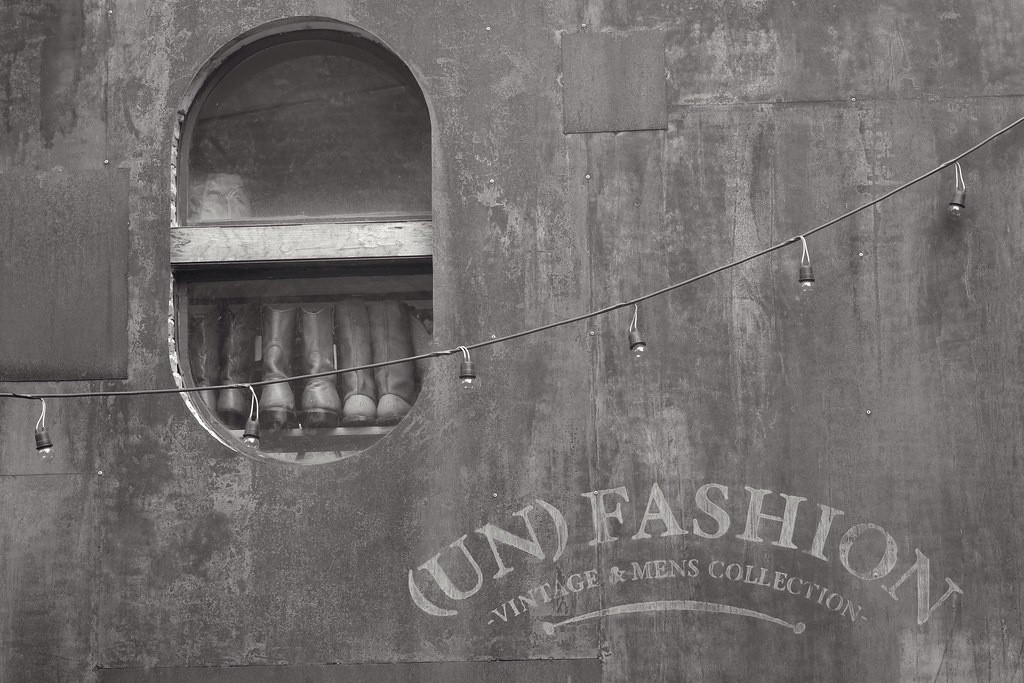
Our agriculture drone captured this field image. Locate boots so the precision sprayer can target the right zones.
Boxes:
[409,310,431,389]
[367,299,418,426]
[216,304,256,430]
[296,303,341,428]
[332,298,378,427]
[188,312,218,418]
[257,304,298,429]
